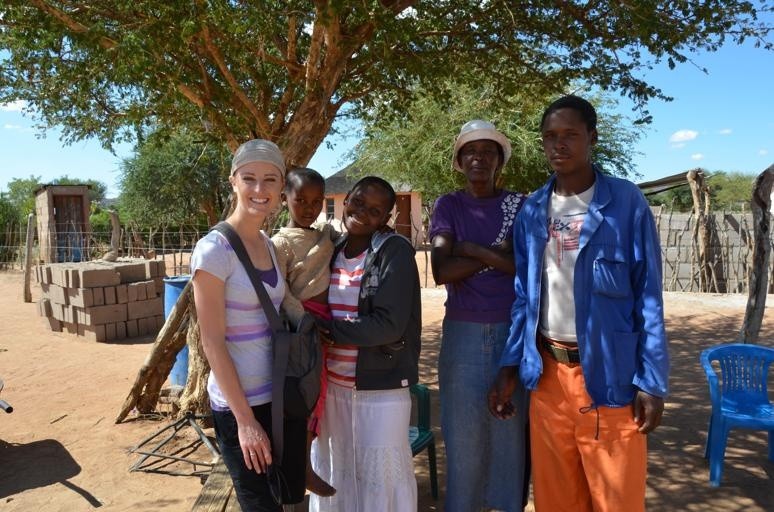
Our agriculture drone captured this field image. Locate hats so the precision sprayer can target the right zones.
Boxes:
[452,119,512,174]
[231,139,287,181]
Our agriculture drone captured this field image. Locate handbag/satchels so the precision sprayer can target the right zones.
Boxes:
[273,311,322,421]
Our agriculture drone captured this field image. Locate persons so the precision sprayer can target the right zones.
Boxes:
[424,115,534,512]
[270,166,394,498]
[484,95,671,510]
[287,173,420,510]
[188,139,303,512]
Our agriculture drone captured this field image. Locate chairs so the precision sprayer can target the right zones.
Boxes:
[700,342,774,487]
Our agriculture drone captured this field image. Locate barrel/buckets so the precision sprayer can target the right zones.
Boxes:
[162,277,192,386]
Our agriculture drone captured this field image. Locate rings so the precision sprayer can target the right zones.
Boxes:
[249,452,257,457]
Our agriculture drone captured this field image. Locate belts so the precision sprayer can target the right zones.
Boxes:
[542,341,579,364]
